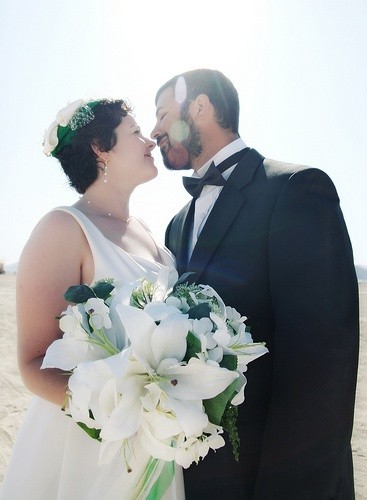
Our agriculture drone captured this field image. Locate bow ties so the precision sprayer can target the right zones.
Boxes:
[181,147,251,199]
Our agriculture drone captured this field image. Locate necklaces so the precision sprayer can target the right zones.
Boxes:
[79,196,131,221]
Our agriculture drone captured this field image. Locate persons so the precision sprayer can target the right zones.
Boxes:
[150,67,361,500]
[0,97,187,500]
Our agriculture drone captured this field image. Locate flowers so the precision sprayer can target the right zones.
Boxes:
[39,270,269,500]
